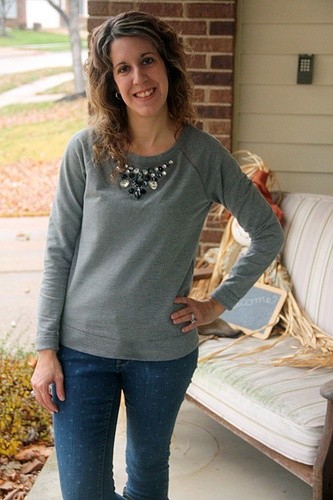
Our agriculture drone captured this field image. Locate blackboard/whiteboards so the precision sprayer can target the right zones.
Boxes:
[212,273,288,340]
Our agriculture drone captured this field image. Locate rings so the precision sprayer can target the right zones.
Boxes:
[191,314,196,324]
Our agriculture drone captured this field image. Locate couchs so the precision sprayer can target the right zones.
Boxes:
[185,190,333,500]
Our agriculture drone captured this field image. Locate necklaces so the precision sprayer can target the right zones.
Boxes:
[117,155,175,200]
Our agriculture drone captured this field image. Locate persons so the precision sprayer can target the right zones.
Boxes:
[29,10,286,500]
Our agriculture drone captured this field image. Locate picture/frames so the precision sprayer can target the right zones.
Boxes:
[219,282,288,339]
